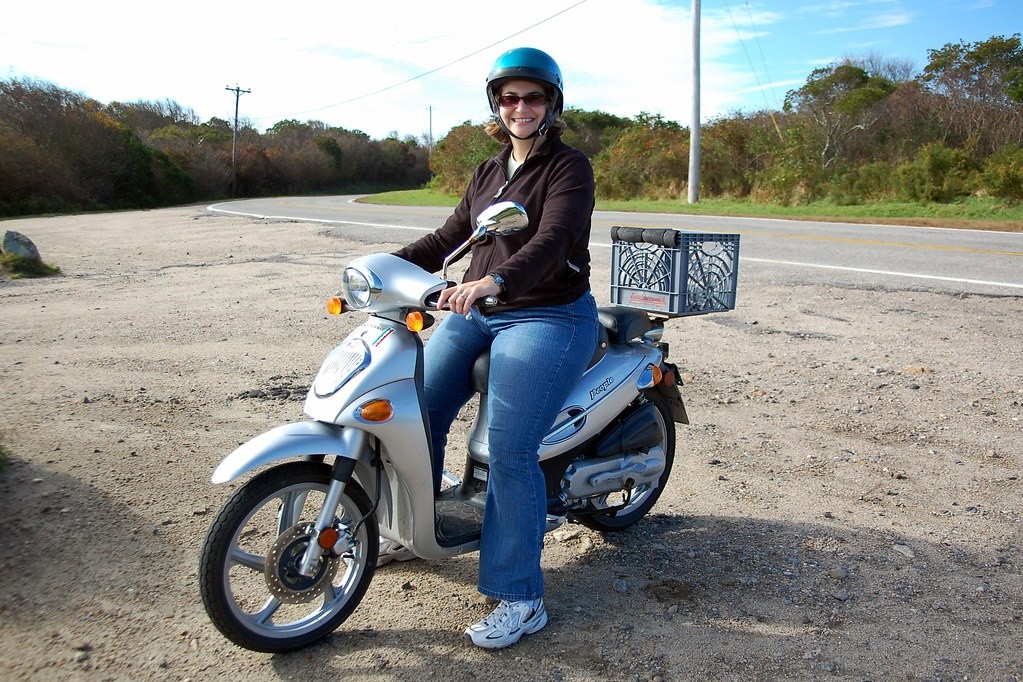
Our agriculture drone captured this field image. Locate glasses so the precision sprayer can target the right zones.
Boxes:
[494,94,550,107]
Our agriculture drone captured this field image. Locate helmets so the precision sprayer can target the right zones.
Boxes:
[486,47,564,117]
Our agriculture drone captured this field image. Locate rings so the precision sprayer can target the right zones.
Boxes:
[461,294,466,299]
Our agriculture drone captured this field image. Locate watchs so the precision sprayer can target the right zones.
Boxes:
[489,273,505,293]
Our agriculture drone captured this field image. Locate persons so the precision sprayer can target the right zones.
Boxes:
[337,47,599,648]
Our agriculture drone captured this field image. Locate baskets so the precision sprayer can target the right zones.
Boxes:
[609,224,740,318]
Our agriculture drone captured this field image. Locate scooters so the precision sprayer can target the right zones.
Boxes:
[192,199,692,656]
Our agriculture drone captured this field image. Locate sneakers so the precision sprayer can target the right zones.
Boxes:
[464,599,548,649]
[341,535,419,569]
